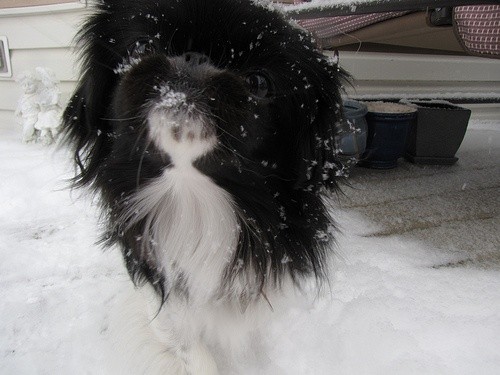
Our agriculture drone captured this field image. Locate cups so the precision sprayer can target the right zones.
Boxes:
[331,98,368,169]
[359,98,416,168]
[399,98,472,166]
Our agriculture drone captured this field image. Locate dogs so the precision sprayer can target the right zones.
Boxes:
[44,0,361,375]
